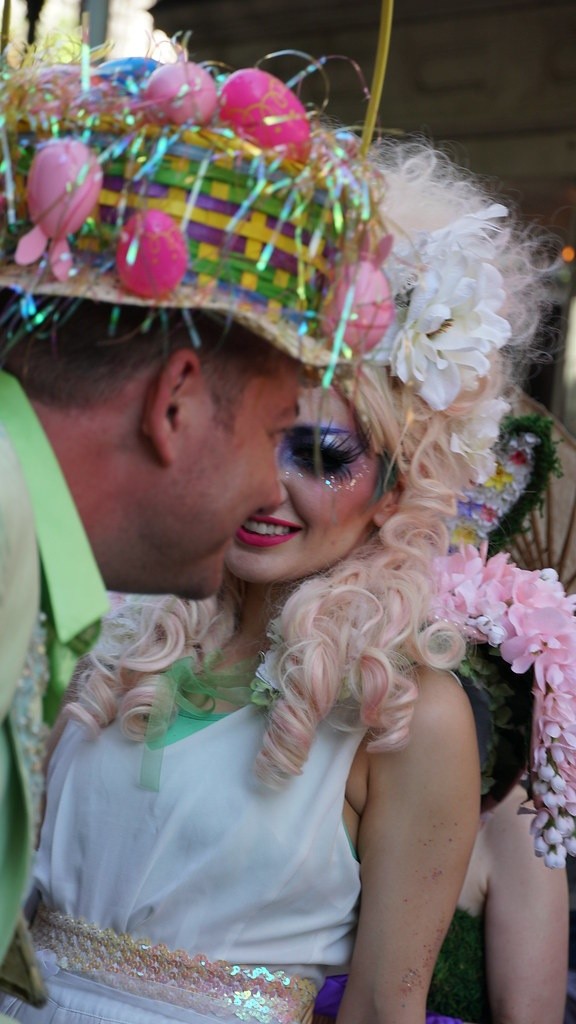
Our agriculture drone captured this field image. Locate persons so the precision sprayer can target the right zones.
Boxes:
[0,53,392,1024]
[0,153,576,1024]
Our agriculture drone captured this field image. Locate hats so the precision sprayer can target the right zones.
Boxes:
[0,26,387,387]
